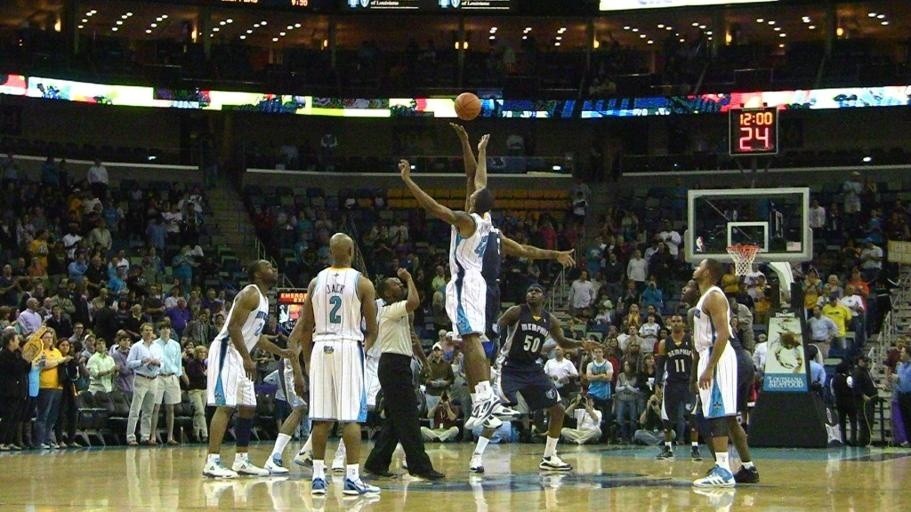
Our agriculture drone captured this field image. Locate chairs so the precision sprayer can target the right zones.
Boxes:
[70,394,283,444]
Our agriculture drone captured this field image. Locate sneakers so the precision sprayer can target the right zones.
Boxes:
[202,463,239,481]
[294,450,348,474]
[468,460,485,473]
[231,459,270,477]
[311,478,329,494]
[264,455,289,474]
[484,405,519,431]
[693,464,734,489]
[656,445,704,463]
[463,395,502,429]
[202,461,203,463]
[342,478,381,496]
[538,456,573,471]
[735,465,759,483]
[1,439,85,451]
[128,437,209,445]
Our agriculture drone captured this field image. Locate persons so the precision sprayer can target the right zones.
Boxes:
[0,10,911,497]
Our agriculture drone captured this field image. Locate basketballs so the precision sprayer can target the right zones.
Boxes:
[455,93,482,121]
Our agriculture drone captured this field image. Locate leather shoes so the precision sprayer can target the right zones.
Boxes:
[409,468,447,482]
[363,465,397,480]
